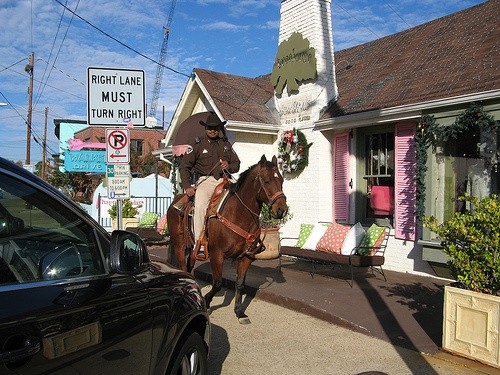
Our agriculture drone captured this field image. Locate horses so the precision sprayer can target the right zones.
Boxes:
[166,154,289,325]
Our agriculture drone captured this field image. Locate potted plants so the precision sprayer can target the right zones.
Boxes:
[240,201,294,260]
[418,190,500,371]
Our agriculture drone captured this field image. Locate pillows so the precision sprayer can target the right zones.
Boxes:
[157,214,168,235]
[315,222,353,255]
[137,211,161,229]
[356,222,387,257]
[340,222,367,255]
[294,224,323,248]
[300,223,329,252]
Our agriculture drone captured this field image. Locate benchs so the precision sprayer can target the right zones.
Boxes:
[125,210,170,248]
[278,222,391,286]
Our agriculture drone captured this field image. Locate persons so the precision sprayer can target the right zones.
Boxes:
[465,158,492,212]
[178,114,241,259]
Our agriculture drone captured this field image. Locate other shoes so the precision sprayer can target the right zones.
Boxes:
[197,251,205,258]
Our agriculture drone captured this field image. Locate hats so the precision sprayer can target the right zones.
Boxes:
[199,111,227,128]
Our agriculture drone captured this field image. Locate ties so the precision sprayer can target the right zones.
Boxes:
[211,141,220,181]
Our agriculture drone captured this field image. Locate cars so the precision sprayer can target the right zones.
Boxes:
[0,156,210,375]
